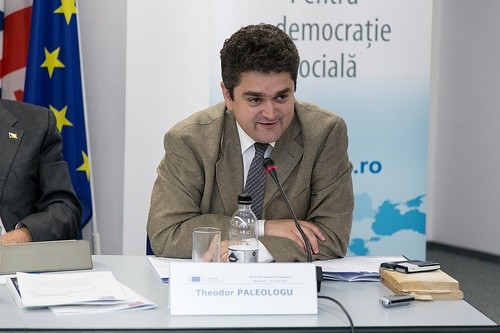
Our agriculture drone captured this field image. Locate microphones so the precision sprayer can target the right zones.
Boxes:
[263,156,323,293]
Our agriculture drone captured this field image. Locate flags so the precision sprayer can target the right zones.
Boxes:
[0,0,93,230]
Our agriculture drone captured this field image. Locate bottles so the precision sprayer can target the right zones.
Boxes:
[228,194,260,263]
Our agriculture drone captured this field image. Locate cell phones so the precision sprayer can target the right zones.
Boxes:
[380,294,414,308]
[396,261,441,273]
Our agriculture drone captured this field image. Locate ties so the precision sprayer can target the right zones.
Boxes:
[245,142,270,220]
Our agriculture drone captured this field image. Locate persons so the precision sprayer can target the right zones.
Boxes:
[0,98,83,244]
[146,24,355,263]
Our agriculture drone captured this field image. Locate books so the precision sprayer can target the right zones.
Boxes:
[379,267,464,300]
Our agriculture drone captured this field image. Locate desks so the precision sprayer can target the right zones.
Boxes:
[0,253,500,333]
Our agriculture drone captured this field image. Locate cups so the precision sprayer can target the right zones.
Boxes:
[192,227,221,262]
